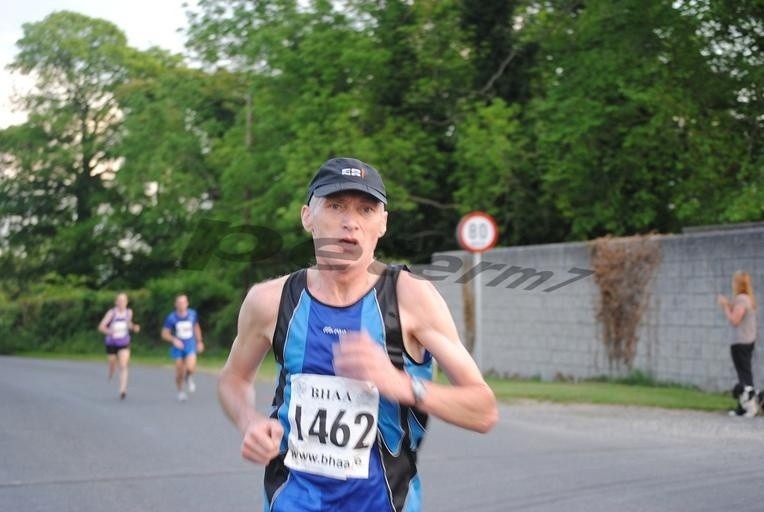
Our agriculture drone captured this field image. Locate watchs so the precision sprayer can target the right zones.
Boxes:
[410,374,428,408]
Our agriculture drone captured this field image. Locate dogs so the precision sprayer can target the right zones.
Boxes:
[727,383,764,418]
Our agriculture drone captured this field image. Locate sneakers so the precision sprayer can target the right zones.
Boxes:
[178,392,186,398]
[185,381,196,392]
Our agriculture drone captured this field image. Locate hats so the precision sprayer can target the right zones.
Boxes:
[307,158,387,210]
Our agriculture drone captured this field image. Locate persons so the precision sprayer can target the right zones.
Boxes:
[98,292,140,401]
[717,270,758,418]
[160,292,204,401]
[216,155,500,512]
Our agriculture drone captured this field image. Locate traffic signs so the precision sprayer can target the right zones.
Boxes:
[456,211,498,253]
